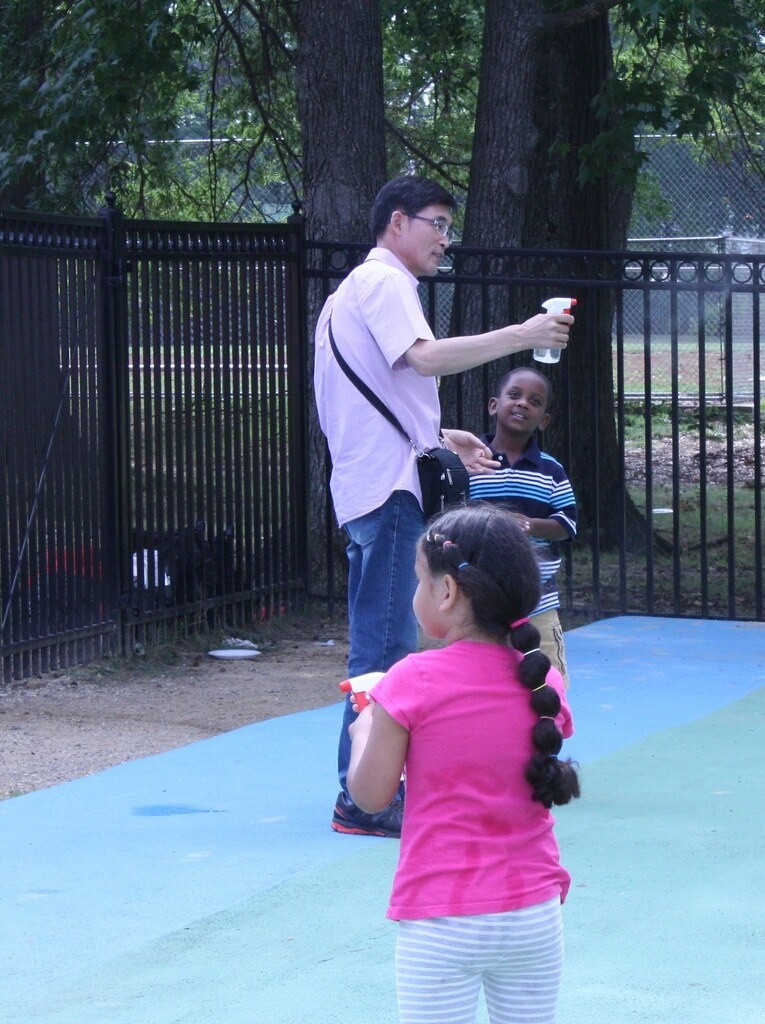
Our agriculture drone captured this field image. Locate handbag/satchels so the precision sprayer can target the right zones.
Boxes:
[417,449,472,521]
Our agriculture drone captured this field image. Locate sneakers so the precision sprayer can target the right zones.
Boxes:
[330,791,406,838]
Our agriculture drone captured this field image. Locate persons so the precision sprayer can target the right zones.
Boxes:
[312,171,574,838]
[344,504,571,1024]
[468,366,576,739]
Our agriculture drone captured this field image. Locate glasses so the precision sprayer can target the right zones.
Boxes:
[386,212,456,247]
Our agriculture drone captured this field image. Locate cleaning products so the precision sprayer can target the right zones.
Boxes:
[528,295,578,365]
[336,669,390,714]
